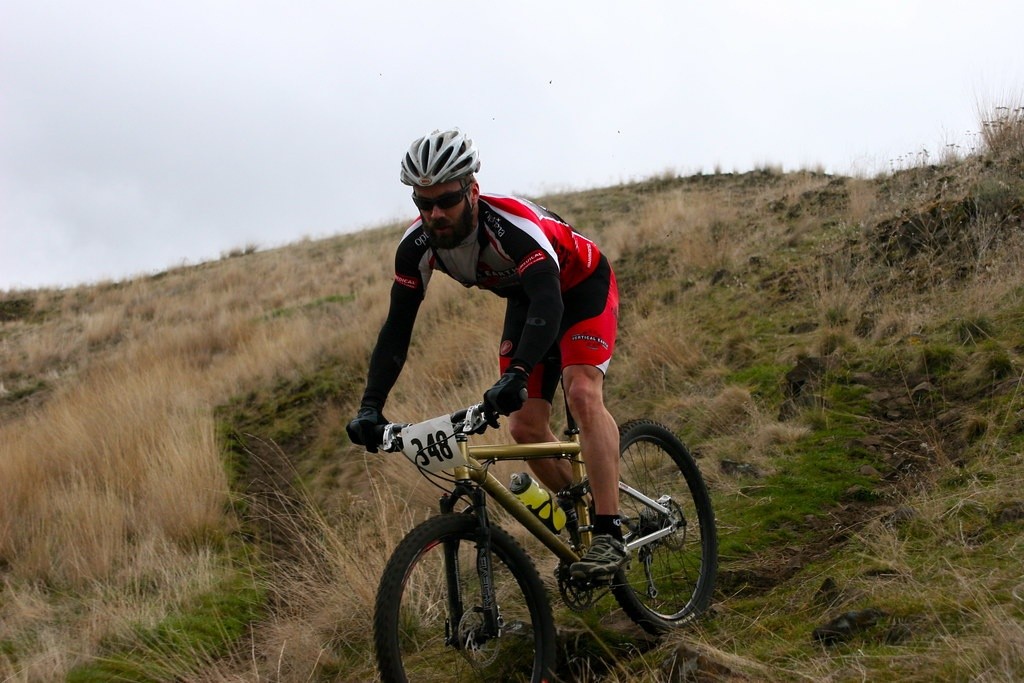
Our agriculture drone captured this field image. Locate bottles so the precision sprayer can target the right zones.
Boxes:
[509,471,565,532]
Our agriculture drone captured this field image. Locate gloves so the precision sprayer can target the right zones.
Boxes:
[346,407,389,453]
[482,367,529,429]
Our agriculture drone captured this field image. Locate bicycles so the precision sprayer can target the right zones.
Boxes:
[347,386,718,683]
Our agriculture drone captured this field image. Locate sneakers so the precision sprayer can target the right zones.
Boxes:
[553,537,573,577]
[570,533,633,578]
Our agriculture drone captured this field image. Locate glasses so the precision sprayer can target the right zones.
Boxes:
[411,182,472,211]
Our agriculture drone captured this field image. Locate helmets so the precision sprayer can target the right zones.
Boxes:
[400,129,480,190]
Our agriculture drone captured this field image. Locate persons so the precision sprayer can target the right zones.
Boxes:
[347,128,629,582]
